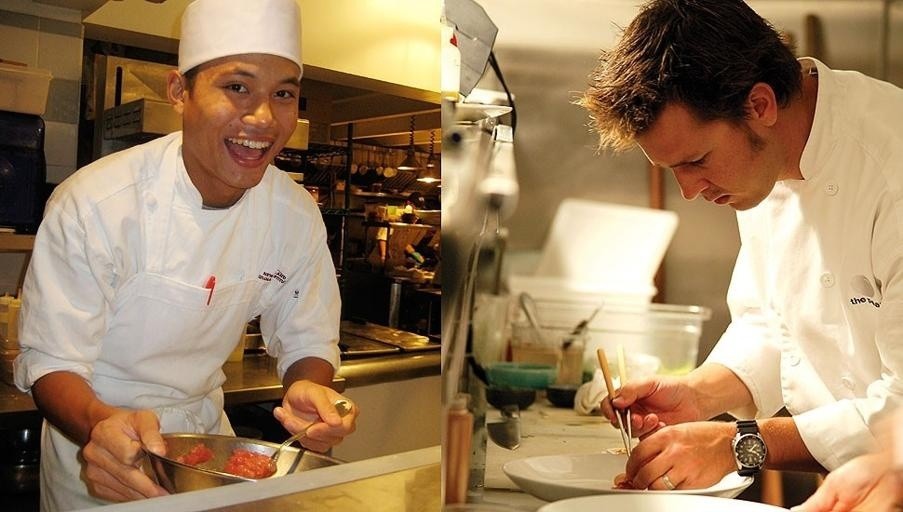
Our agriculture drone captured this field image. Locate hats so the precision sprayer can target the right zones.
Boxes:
[178,0,304,81]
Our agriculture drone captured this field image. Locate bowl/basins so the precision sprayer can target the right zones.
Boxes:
[545,384,578,410]
[485,383,537,413]
[140,432,351,499]
[506,291,712,377]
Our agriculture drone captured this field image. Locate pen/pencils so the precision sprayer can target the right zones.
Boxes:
[206,276,215,306]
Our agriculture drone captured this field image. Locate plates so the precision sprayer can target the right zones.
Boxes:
[501,453,756,501]
[534,494,791,512]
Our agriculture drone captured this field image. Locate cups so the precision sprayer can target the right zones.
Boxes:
[511,321,593,385]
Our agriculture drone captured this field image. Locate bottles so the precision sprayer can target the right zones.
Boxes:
[2,289,22,373]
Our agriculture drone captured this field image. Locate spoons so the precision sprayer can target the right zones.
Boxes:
[230,399,355,479]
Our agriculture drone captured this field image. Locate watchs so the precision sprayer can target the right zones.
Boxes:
[730,420,767,477]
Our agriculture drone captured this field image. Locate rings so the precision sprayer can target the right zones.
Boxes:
[657,469,678,491]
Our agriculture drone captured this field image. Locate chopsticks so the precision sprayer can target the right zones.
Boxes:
[590,345,635,464]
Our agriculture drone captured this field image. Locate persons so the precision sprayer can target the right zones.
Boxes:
[568,2,901,492]
[4,0,370,510]
[779,446,902,511]
[366,191,427,263]
